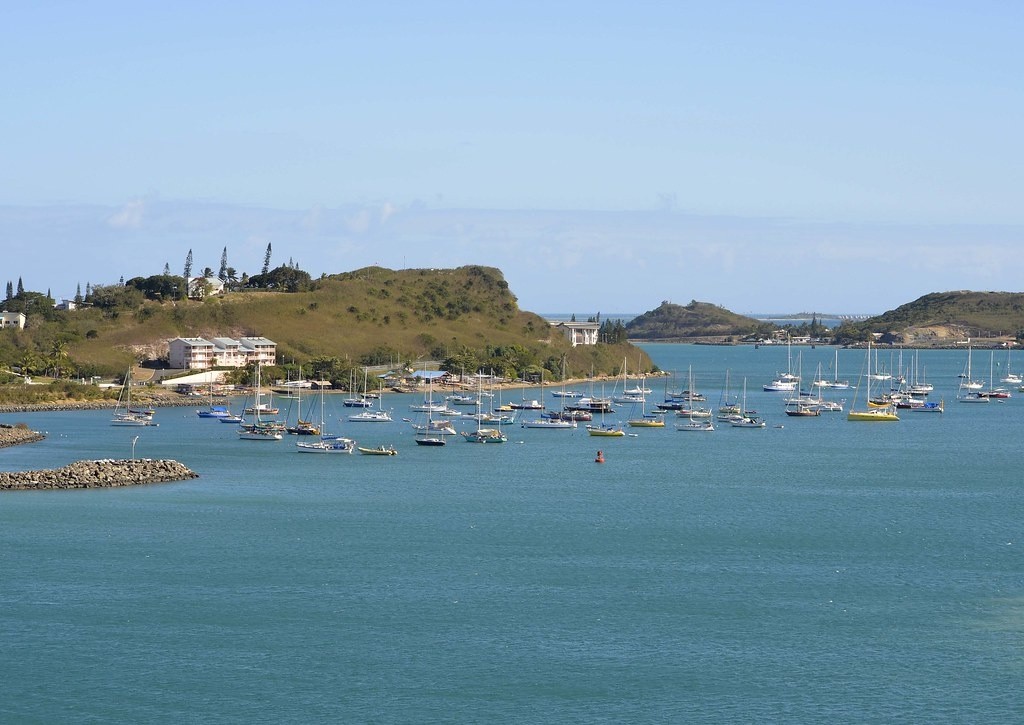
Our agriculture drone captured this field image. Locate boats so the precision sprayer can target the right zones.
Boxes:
[357,446,399,456]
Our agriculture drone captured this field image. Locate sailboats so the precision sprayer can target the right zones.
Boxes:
[403,359,757,444]
[761,330,1023,423]
[195,344,394,454]
[729,377,765,428]
[109,360,160,427]
